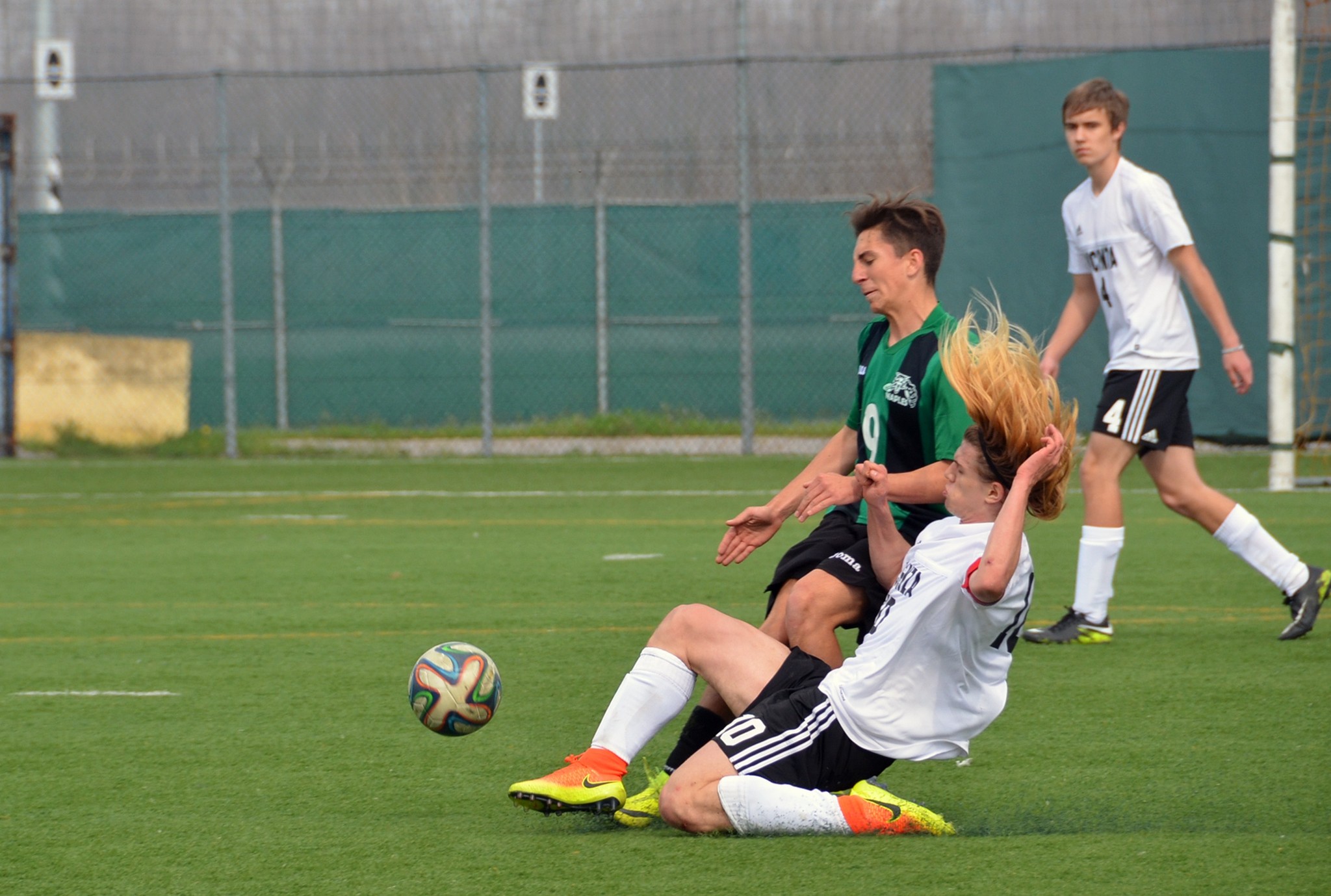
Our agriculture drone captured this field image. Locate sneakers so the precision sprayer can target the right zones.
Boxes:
[1022,604,1113,644]
[609,757,670,828]
[850,775,958,836]
[507,752,627,817]
[1277,564,1331,641]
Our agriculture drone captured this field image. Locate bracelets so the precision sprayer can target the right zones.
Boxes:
[1224,344,1245,354]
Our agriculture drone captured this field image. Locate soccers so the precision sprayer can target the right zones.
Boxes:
[407,642,499,737]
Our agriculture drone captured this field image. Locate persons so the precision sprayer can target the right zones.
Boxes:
[507,294,1078,837]
[615,188,979,827]
[1020,75,1331,646]
